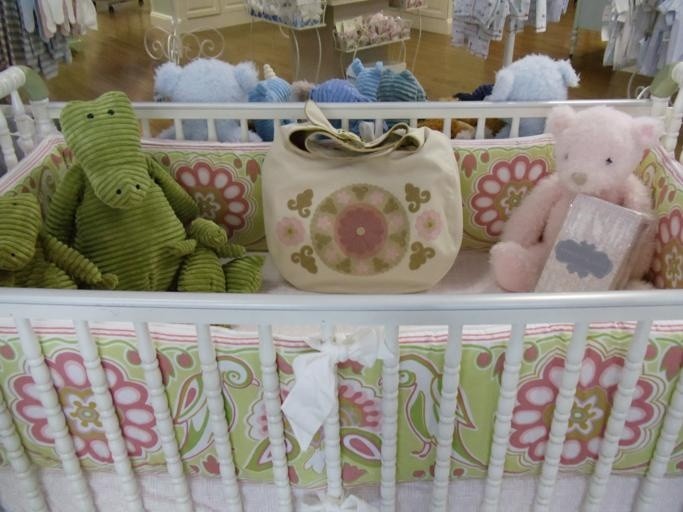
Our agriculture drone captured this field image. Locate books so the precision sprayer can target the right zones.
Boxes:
[531,190,652,292]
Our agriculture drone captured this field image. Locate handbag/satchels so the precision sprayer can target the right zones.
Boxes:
[261,99,463,294]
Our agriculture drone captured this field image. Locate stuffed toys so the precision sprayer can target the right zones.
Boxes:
[252,59,426,140]
[151,56,259,143]
[40,89,264,294]
[0,191,99,292]
[486,98,673,294]
[162,217,263,293]
[452,52,581,139]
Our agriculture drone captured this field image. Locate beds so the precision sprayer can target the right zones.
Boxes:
[0,132,681,512]
